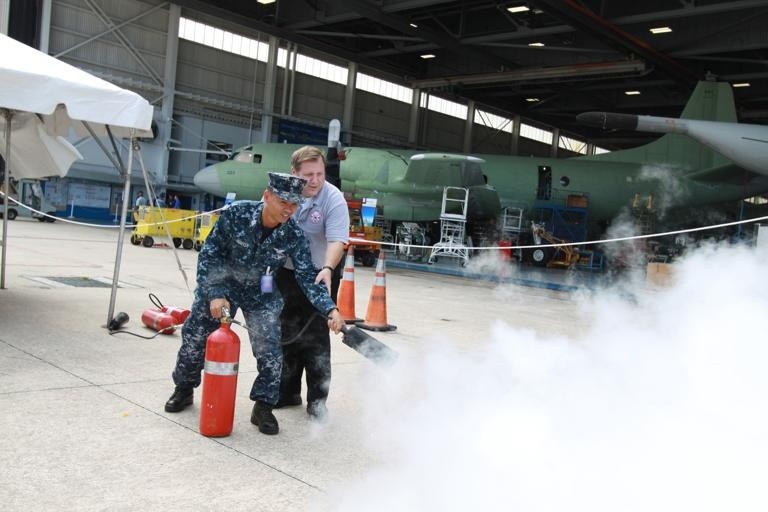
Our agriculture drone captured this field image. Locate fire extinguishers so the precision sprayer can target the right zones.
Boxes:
[199,307,240,438]
[141,306,190,335]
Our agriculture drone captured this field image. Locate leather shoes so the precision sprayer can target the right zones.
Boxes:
[251,400,279,433]
[166,385,193,411]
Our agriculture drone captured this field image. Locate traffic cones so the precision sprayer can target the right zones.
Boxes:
[336,246,367,323]
[347,250,397,332]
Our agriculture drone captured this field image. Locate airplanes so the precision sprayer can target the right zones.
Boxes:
[191,73,767,266]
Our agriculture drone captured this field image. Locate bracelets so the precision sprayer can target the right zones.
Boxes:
[322,266,334,271]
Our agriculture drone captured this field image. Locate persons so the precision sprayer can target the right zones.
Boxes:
[259,147,350,423]
[132,190,181,232]
[164,171,345,434]
[30,178,43,218]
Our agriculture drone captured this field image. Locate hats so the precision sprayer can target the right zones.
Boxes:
[268,172,307,204]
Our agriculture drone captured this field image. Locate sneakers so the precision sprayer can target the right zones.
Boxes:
[273,393,302,408]
[307,402,327,418]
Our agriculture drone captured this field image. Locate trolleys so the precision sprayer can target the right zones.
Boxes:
[395,227,426,263]
